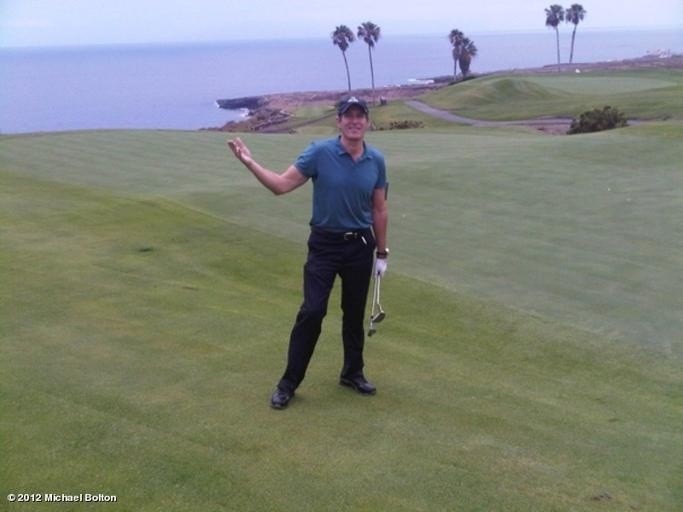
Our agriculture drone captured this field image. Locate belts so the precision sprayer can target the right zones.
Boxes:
[311,227,358,240]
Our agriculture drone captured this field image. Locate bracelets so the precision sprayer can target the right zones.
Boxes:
[375,247,388,258]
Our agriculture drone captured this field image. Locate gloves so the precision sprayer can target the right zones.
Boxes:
[374,258,388,279]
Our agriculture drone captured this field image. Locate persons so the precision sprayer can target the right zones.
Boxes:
[227,95,390,409]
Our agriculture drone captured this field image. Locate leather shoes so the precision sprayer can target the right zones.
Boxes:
[339,375,377,396]
[270,385,295,410]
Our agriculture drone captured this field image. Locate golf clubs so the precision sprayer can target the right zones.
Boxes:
[368,273,384,336]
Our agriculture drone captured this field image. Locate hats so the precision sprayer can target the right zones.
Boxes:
[337,96,369,114]
